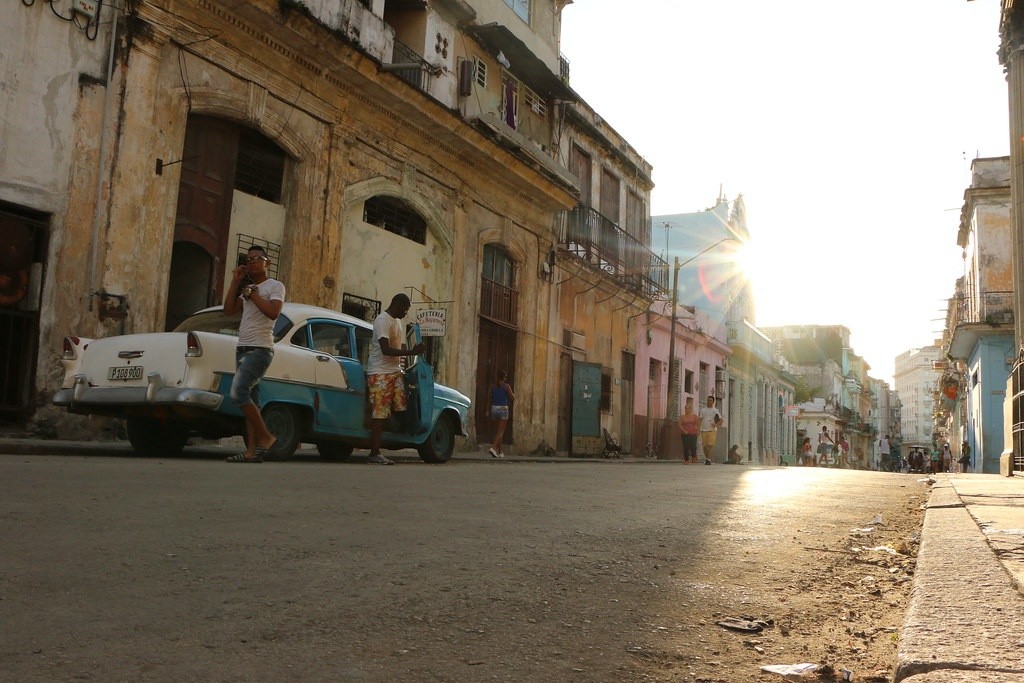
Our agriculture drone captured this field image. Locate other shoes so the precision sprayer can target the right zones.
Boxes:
[692,458,696,464]
[683,460,689,464]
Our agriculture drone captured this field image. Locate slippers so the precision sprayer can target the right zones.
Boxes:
[250,439,282,462]
[227,454,264,462]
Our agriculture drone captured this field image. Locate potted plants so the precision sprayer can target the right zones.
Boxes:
[933,411,942,425]
[941,371,959,399]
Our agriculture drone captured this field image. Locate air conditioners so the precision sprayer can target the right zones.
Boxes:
[716,371,726,382]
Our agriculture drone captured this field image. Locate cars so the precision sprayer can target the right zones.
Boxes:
[52,301,471,462]
[814,441,839,465]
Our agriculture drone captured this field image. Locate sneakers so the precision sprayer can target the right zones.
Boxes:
[705,458,711,465]
[489,448,498,458]
[367,452,395,465]
[499,451,504,458]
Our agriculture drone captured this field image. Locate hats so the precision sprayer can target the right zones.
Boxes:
[963,440,969,443]
[944,444,948,446]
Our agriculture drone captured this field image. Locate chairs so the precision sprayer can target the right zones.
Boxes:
[779,455,788,466]
[601,428,623,460]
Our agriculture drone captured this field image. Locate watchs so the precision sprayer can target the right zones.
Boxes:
[244,285,255,301]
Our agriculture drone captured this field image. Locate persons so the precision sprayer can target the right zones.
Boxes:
[223,245,285,463]
[485,369,515,458]
[366,293,425,465]
[799,425,865,470]
[729,444,741,464]
[679,395,724,464]
[879,435,972,473]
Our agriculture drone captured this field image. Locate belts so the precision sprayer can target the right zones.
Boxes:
[237,346,274,352]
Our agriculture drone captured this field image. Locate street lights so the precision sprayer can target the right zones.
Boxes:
[666,237,743,424]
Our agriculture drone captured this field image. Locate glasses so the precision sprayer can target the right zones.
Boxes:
[246,255,268,261]
